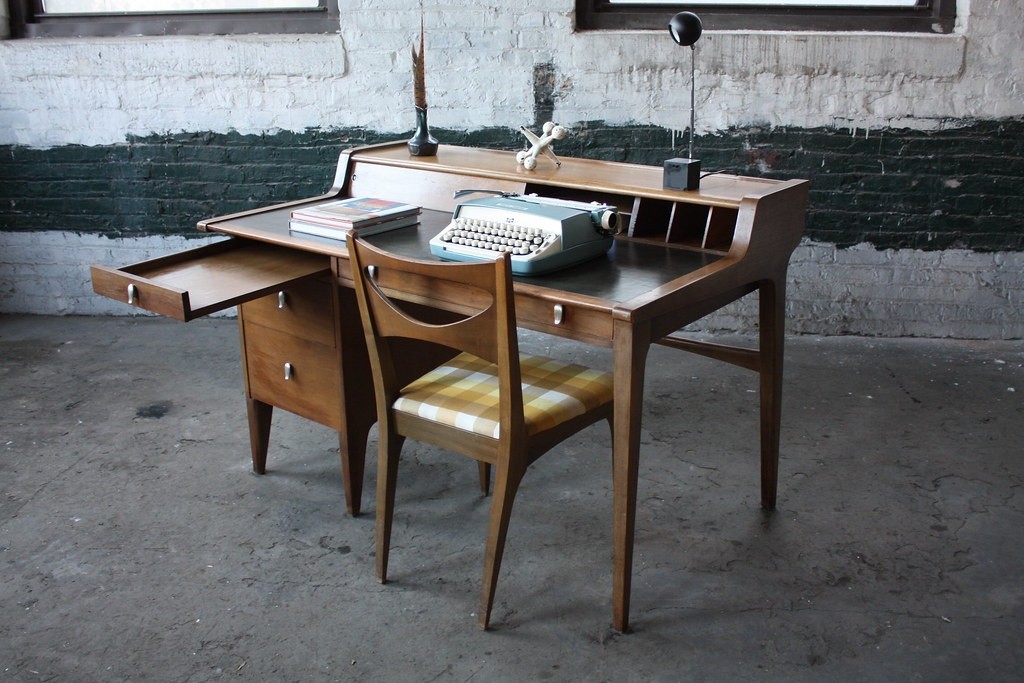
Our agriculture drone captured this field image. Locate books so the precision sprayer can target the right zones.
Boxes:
[288,196,423,242]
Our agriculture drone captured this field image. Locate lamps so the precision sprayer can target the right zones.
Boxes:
[662,11,703,191]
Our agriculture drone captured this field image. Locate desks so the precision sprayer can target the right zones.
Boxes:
[92,139,811,637]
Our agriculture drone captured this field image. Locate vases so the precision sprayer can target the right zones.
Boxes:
[408,104,439,157]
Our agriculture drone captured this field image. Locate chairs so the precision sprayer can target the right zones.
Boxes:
[345,229,613,632]
